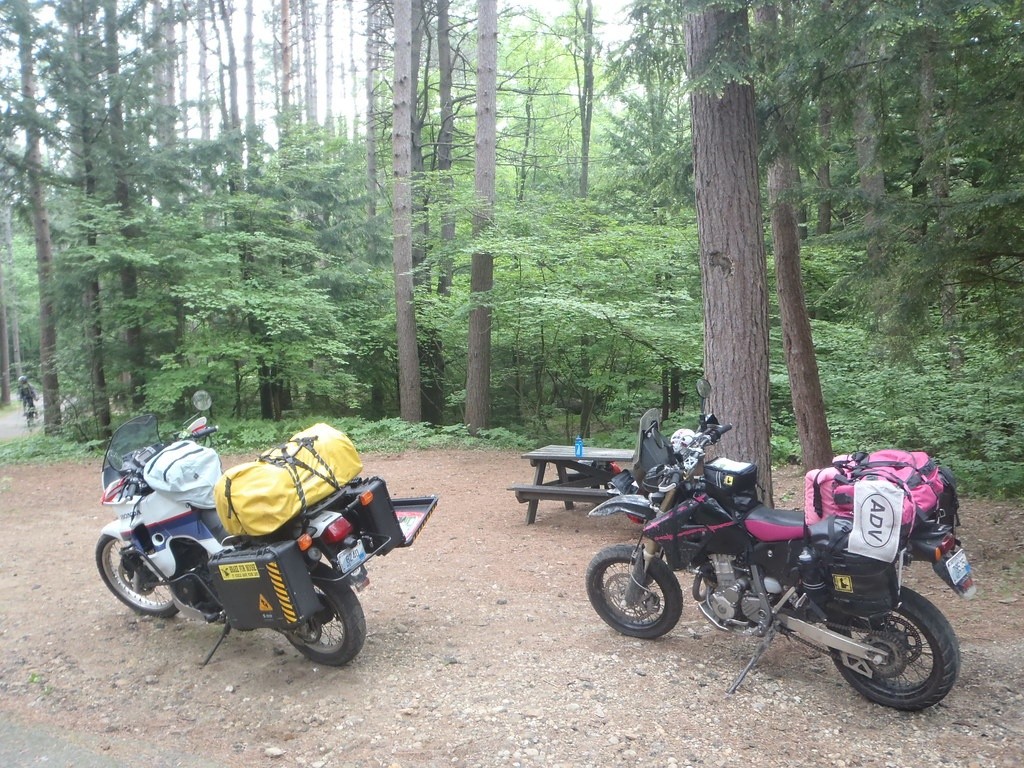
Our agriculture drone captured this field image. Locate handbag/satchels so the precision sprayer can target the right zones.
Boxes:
[804,441,946,553]
[141,440,224,509]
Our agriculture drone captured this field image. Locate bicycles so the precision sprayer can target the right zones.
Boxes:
[20,396,39,429]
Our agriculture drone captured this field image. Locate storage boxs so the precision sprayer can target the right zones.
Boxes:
[346,478,438,558]
[210,539,322,630]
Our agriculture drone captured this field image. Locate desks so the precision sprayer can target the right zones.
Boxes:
[521,444,637,526]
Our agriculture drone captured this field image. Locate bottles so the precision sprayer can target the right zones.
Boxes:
[575,437,583,458]
[799,549,811,568]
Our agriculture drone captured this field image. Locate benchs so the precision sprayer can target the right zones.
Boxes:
[507,484,620,503]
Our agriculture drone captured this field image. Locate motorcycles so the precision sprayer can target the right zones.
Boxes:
[81,390,440,669]
[584,408,972,712]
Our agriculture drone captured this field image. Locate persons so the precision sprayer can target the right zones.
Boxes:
[17,376,39,417]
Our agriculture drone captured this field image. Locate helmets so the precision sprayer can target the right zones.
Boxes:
[670,428,696,456]
